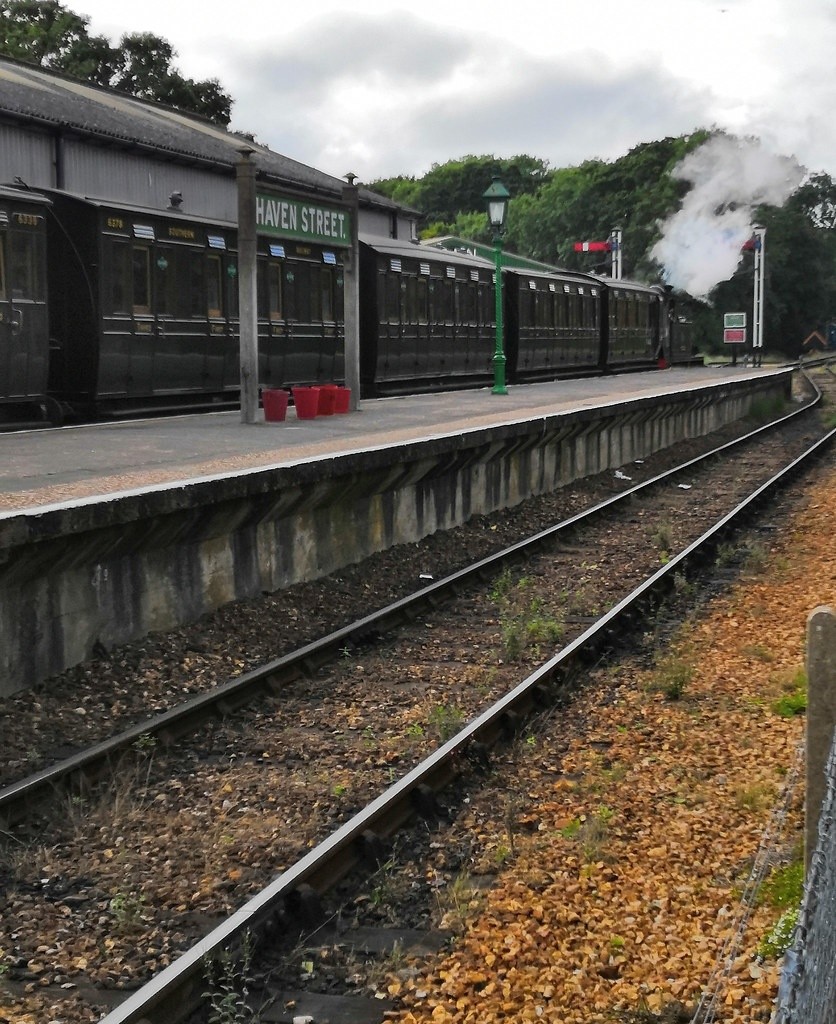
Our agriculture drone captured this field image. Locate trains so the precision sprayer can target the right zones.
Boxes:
[0,176,703,424]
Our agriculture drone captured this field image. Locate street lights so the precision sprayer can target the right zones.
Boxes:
[481,174,512,395]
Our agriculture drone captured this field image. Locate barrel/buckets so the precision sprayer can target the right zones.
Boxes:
[335,387,351,413]
[292,385,319,420]
[262,389,290,421]
[311,385,338,416]
